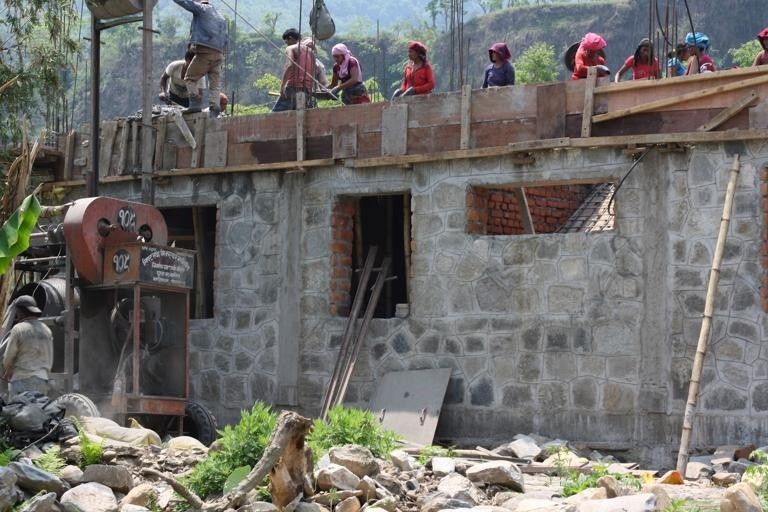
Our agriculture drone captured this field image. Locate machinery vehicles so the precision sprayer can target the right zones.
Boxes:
[1,196,217,447]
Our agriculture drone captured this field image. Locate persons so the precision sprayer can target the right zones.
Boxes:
[202,93,229,119]
[325,43,371,106]
[481,42,514,88]
[159,50,206,108]
[302,37,327,107]
[664,43,690,78]
[571,31,609,76]
[172,0,229,119]
[2,295,54,400]
[752,27,768,67]
[391,41,434,99]
[614,38,660,83]
[271,28,315,112]
[683,31,718,76]
